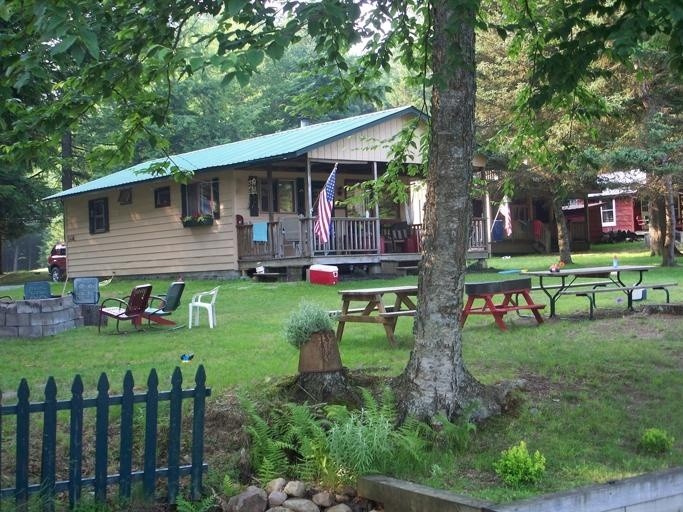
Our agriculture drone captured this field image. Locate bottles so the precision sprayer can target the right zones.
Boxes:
[613,255,618,268]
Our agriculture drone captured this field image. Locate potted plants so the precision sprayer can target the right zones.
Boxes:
[286,298,344,373]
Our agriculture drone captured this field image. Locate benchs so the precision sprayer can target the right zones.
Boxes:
[493,305,546,330]
[559,282,678,320]
[327,305,395,342]
[379,310,418,348]
[516,279,621,313]
[462,305,504,333]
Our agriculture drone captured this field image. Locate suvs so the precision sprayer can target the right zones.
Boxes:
[48,242,67,283]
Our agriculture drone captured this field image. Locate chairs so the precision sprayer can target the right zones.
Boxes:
[188,286,220,329]
[98,284,152,333]
[135,282,185,329]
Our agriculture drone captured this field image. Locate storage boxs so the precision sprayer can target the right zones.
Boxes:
[309,264,339,285]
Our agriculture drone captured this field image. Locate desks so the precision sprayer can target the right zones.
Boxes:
[336,285,418,348]
[461,278,544,330]
[519,265,648,317]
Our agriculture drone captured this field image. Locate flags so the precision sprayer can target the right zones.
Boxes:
[314,167,337,244]
[500,201,512,236]
